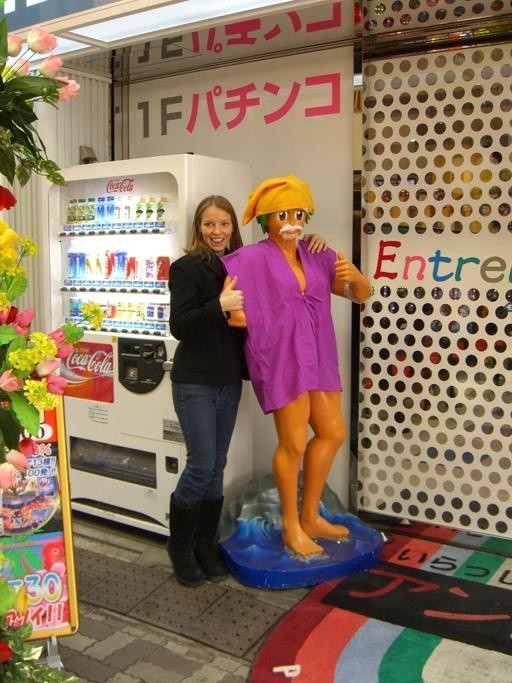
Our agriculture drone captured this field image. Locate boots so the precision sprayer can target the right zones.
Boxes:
[167,491,206,587]
[193,494,230,583]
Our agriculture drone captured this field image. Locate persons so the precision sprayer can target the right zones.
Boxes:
[167,193,250,588]
[217,173,374,557]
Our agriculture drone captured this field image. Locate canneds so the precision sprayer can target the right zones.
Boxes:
[157,256,170,280]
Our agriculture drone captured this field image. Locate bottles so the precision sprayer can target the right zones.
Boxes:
[61,189,174,339]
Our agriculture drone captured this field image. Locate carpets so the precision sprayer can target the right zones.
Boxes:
[246,531,512,683]
